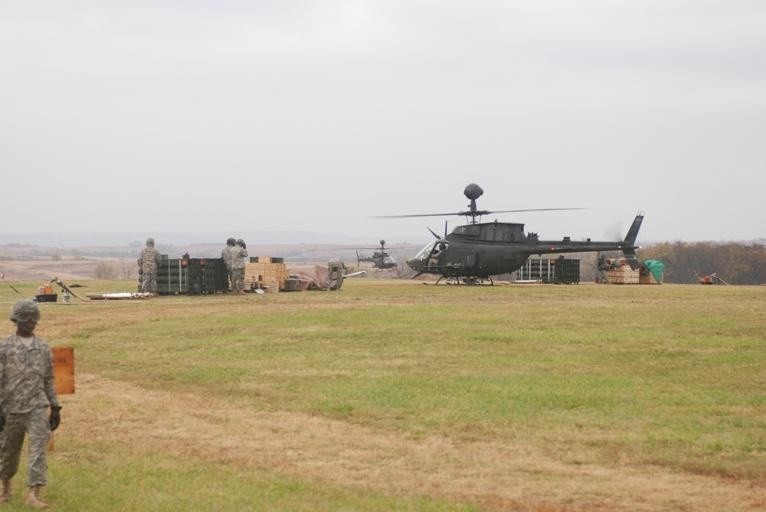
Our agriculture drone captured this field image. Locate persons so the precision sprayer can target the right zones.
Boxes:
[230,239,249,295]
[222,238,236,276]
[0,300,62,510]
[140,237,163,296]
[426,243,446,270]
[596,251,611,284]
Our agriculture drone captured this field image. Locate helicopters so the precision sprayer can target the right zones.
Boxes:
[355,240,398,273]
[377,183,644,286]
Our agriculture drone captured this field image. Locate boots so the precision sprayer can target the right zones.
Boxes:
[0,479,12,503]
[231,289,238,296]
[238,288,248,295]
[24,484,50,509]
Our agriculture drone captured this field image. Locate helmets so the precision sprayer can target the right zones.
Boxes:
[146,238,154,245]
[236,239,245,248]
[9,302,40,324]
[226,239,235,246]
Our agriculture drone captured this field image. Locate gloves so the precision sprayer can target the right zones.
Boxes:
[0,410,4,432]
[48,405,62,431]
